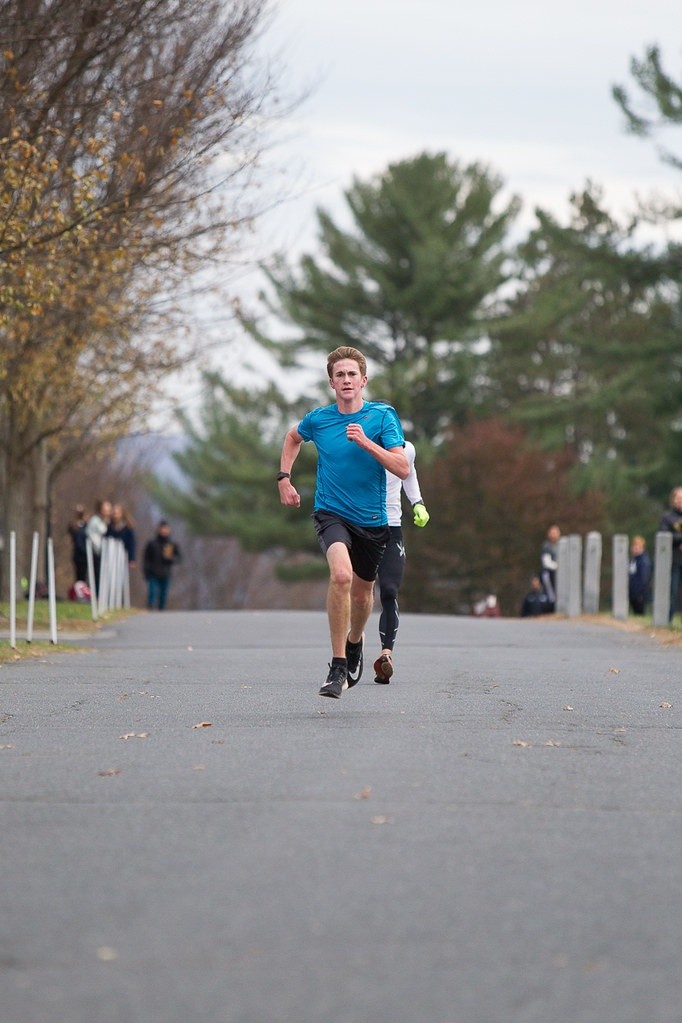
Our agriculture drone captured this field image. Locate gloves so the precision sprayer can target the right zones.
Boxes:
[413,503,430,528]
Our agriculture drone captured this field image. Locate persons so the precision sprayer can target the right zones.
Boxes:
[277,345,410,698]
[67,503,137,605]
[659,487,682,623]
[142,519,181,611]
[370,399,429,684]
[521,525,561,617]
[628,536,650,616]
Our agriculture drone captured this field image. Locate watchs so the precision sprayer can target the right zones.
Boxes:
[278,472,290,482]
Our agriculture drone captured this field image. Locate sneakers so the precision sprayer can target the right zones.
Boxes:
[373,654,394,685]
[318,662,348,699]
[341,628,365,688]
